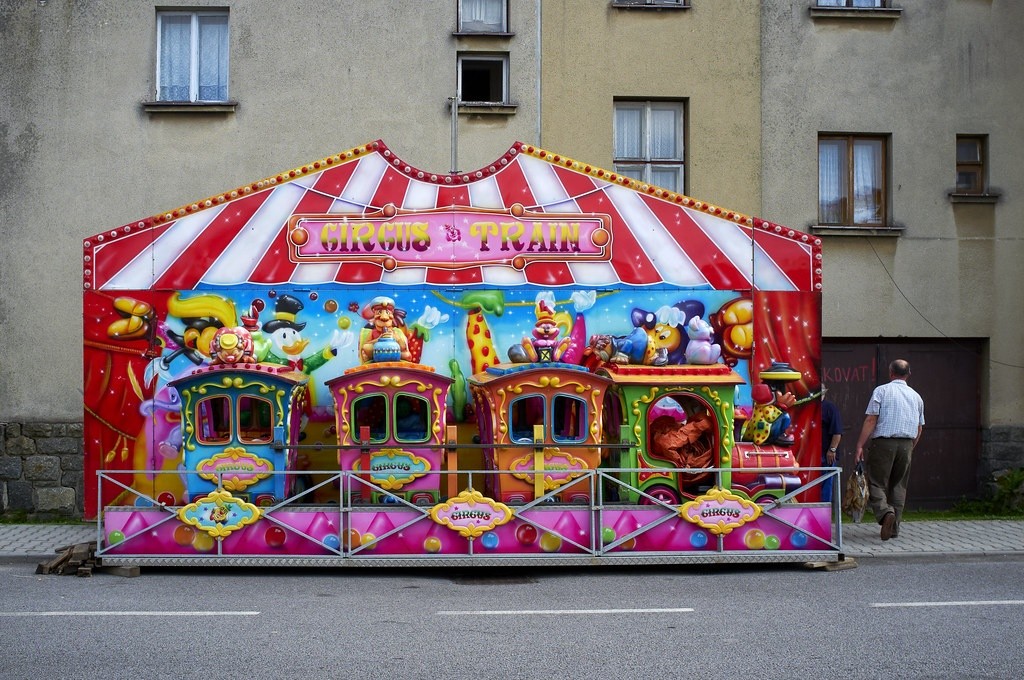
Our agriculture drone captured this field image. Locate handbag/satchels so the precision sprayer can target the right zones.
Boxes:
[842,460,869,525]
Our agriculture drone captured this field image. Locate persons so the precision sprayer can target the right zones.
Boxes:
[854,359,925,540]
[821,382,844,503]
[360,296,413,362]
[207,326,257,367]
[589,326,669,367]
[742,362,802,446]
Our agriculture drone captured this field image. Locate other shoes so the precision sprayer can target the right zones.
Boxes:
[881,513,895,540]
[891,535,898,538]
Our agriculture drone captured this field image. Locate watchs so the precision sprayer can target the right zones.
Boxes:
[829,447,837,452]
